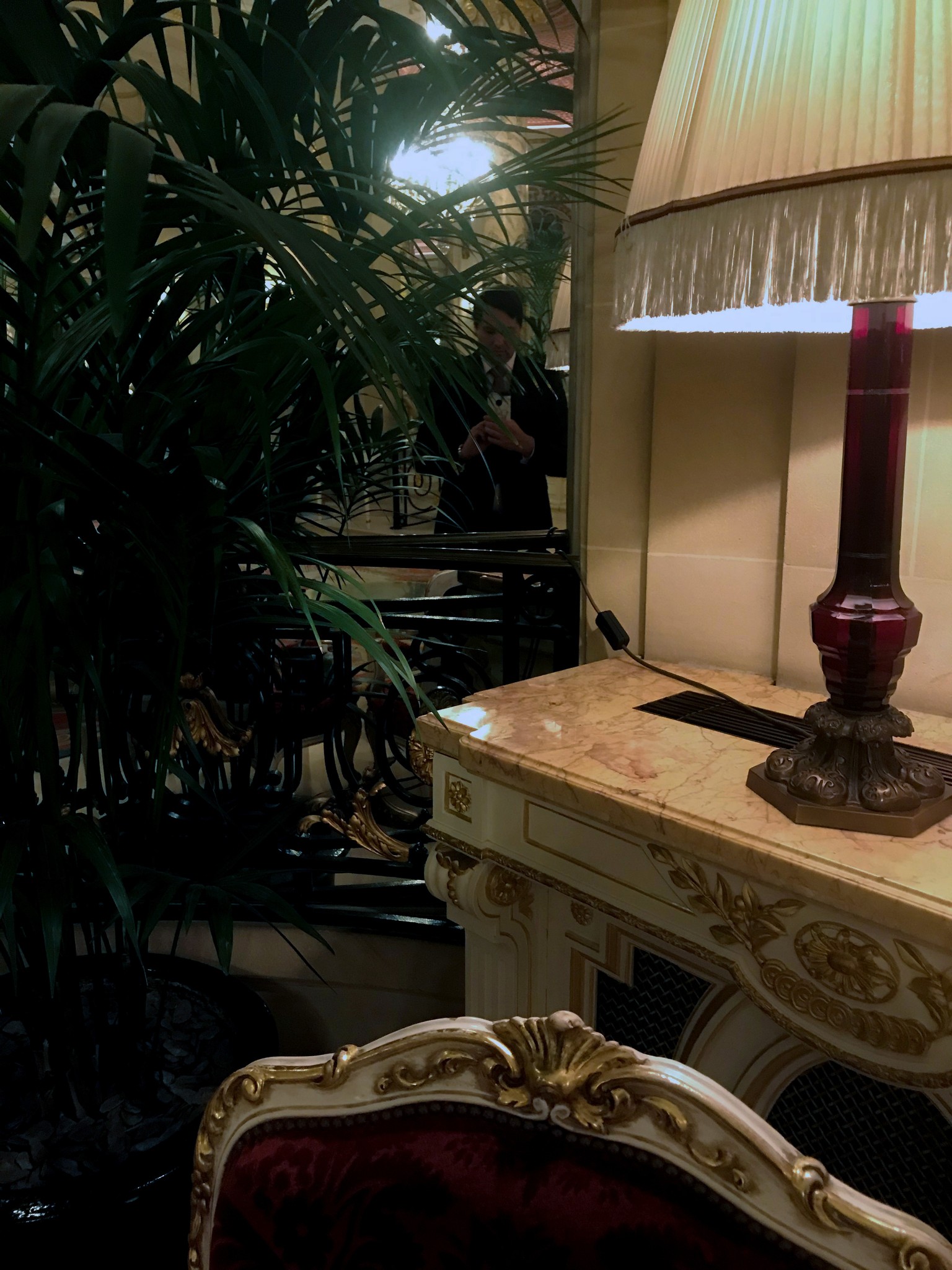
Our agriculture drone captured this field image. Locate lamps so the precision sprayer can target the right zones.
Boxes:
[613,0,952,839]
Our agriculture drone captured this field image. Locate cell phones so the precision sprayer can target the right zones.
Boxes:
[487,395,512,420]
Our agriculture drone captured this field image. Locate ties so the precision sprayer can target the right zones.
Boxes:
[490,364,508,393]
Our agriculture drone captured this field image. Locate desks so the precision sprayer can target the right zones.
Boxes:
[413,656,951,1120]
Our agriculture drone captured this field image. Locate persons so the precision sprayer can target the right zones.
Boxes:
[411,285,570,537]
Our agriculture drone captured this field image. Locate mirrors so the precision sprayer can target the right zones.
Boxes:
[65,1,571,731]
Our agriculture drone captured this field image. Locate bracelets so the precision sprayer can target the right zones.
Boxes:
[458,444,467,460]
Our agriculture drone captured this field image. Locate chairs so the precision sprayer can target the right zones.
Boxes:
[185,1011,951,1270]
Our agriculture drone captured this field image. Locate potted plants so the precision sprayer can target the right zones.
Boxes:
[0,0,649,1270]
